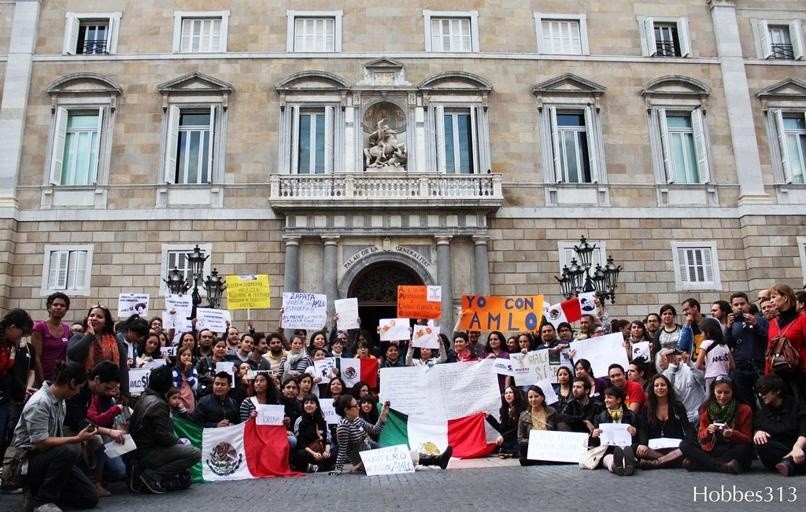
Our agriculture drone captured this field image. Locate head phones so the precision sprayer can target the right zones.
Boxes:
[607,404,623,420]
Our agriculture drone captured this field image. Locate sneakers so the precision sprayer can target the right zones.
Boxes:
[722,459,741,475]
[130,464,167,495]
[682,458,702,472]
[775,459,795,477]
[92,480,112,497]
[0,486,23,495]
[33,502,64,512]
[306,463,319,473]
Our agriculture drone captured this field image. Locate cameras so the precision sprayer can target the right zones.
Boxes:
[79,419,99,432]
[713,423,726,429]
[734,311,744,322]
[385,401,390,406]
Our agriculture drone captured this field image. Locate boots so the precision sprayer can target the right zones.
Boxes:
[635,447,684,471]
[611,445,635,477]
[419,446,452,470]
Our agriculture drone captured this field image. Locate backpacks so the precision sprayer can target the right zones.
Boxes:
[765,313,801,378]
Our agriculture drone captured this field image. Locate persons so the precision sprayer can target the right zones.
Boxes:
[364,118,407,167]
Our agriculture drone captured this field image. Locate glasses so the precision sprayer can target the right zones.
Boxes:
[715,375,733,382]
[756,388,771,396]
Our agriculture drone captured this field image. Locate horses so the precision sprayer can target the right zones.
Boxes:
[363,125,406,167]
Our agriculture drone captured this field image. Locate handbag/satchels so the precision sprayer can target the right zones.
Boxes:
[160,469,193,492]
[1,445,29,483]
